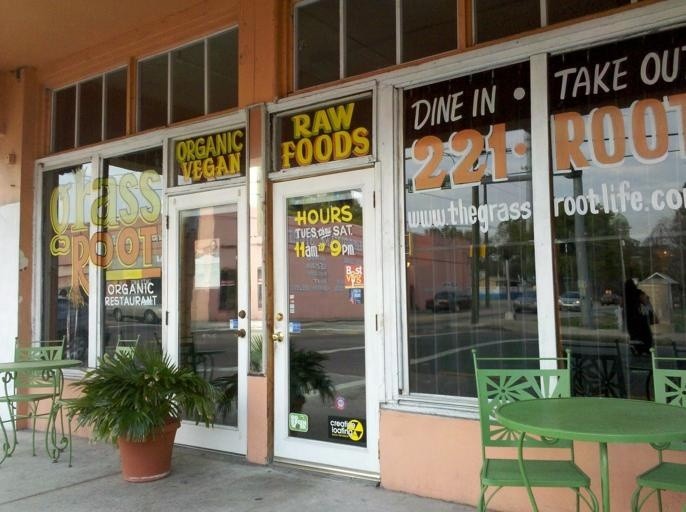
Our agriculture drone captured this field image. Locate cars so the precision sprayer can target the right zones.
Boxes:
[108,293,162,323]
[558,291,593,310]
[600,293,622,305]
[514,291,536,314]
[426,289,471,312]
[55,296,110,361]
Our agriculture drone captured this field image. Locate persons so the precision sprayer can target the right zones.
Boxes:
[624,254,656,356]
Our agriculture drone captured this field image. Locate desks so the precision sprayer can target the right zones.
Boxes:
[0,360,82,463]
[495,396,685,511]
[183,349,227,382]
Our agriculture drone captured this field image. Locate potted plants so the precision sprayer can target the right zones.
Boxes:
[63,336,219,483]
[213,340,339,432]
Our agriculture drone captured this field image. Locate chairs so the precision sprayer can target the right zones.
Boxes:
[153,331,197,378]
[558,339,630,399]
[111,334,141,366]
[471,347,600,511]
[52,334,142,465]
[0,336,67,455]
[633,347,686,511]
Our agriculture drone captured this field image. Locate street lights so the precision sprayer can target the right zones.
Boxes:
[614,228,630,286]
[490,253,518,321]
